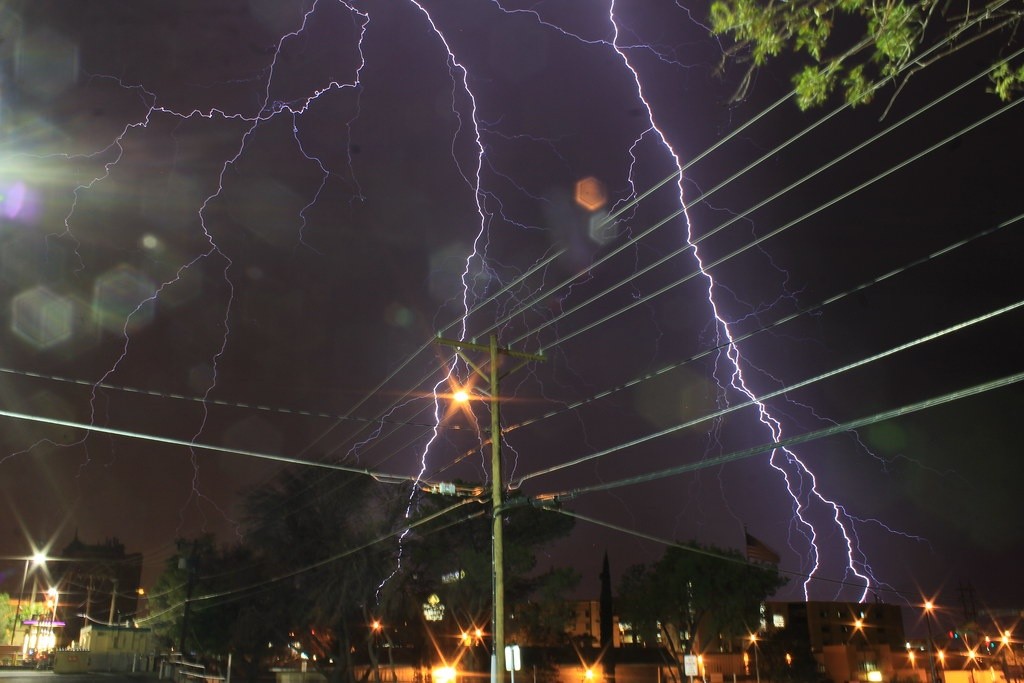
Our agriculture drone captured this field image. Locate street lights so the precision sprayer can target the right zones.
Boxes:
[855,620,870,683]
[751,635,760,683]
[924,601,941,683]
[10,554,47,646]
[45,587,59,670]
